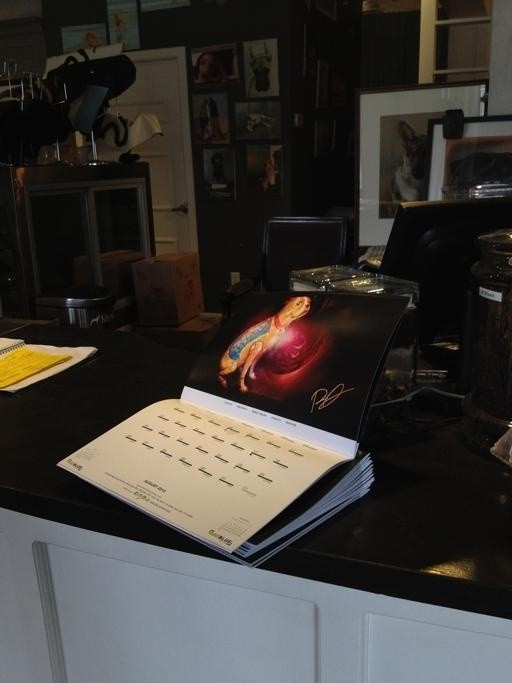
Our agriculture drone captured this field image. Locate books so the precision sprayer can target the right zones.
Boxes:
[0,338,99,395]
[57,386,375,568]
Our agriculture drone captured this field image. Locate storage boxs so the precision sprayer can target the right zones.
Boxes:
[131,251,205,328]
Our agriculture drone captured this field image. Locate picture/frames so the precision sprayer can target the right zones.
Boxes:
[352,80,486,250]
[187,35,282,147]
[55,22,112,55]
[233,144,286,205]
[425,117,512,201]
[104,0,142,51]
[194,149,235,204]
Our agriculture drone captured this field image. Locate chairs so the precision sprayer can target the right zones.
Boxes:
[221,216,346,328]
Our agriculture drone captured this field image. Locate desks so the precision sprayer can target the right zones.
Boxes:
[4,317,512,619]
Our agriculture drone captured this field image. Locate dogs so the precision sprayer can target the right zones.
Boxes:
[217,296,311,392]
[391,119,430,218]
